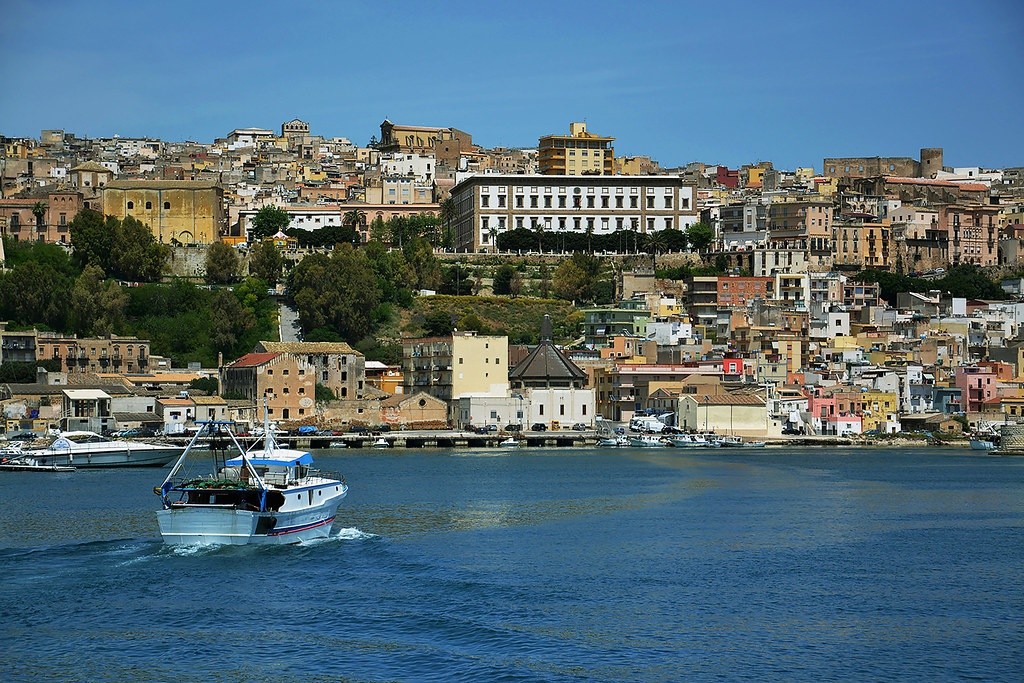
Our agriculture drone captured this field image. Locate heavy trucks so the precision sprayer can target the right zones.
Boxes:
[288,426,318,436]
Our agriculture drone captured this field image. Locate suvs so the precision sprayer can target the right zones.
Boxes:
[532,423,546,431]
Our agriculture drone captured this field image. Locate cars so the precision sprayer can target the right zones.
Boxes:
[661,426,680,434]
[235,431,250,437]
[466,424,497,435]
[573,423,585,430]
[103,427,149,438]
[314,429,343,436]
[612,427,624,433]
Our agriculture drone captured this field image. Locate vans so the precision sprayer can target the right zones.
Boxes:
[349,425,365,432]
[629,418,665,433]
[505,423,523,431]
[374,425,390,432]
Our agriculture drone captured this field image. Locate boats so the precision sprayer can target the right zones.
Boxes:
[500,437,519,449]
[969,419,1002,449]
[596,418,765,448]
[328,440,346,448]
[372,437,390,449]
[0,419,187,468]
[154,389,348,545]
